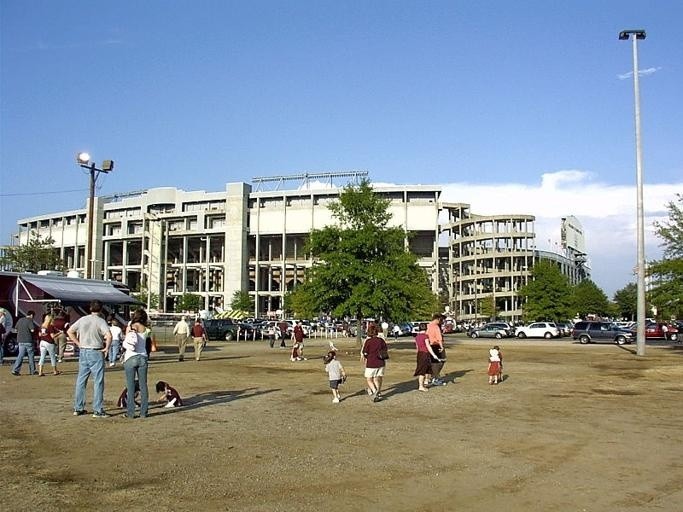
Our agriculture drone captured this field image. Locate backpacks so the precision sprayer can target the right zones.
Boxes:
[122,330,139,351]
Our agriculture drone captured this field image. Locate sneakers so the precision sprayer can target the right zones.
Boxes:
[74,402,175,420]
[332,378,446,404]
[11,359,63,376]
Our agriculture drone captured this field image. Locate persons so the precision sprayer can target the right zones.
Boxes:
[55,311,70,362]
[153,380,182,407]
[171,314,191,362]
[107,315,124,366]
[67,300,113,419]
[37,315,60,377]
[660,324,671,341]
[191,318,208,364]
[11,310,40,374]
[118,380,141,407]
[0,309,6,365]
[123,310,156,418]
[265,309,506,403]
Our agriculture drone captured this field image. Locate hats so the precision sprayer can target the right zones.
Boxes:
[489,349,499,359]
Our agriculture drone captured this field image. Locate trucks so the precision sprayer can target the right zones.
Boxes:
[0,272,131,356]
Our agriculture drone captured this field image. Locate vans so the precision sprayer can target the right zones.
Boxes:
[573,318,682,346]
[468,320,572,340]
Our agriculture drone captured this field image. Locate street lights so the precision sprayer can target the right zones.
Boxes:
[75,152,114,280]
[616,29,653,357]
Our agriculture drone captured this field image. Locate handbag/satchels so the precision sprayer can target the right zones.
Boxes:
[376,348,390,360]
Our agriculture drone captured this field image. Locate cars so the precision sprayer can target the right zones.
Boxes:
[199,315,483,340]
